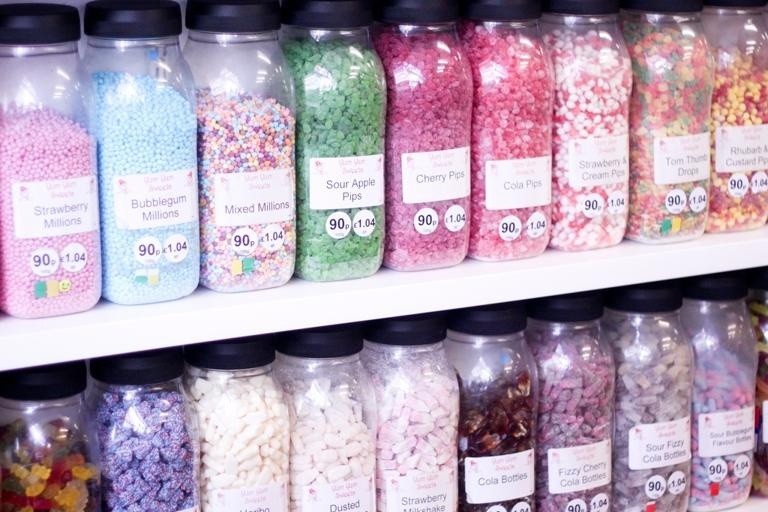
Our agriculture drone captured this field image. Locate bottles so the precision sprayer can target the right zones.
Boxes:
[84,2,198,306]
[2,106,103,317]
[188,339,289,512]
[383,1,471,270]
[0,1,99,315]
[182,1,294,290]
[88,349,200,512]
[555,1,633,247]
[287,0,386,283]
[606,286,692,512]
[360,315,460,512]
[1,362,99,512]
[704,1,764,233]
[681,275,758,511]
[632,1,712,244]
[446,306,538,512]
[276,326,376,512]
[474,1,551,263]
[529,297,614,512]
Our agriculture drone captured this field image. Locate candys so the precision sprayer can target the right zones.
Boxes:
[462,26,553,262]
[541,29,632,251]
[181,367,290,512]
[0,419,102,512]
[707,46,767,233]
[459,372,536,512]
[283,38,384,281]
[281,378,375,512]
[370,365,459,511]
[683,320,754,510]
[198,86,296,294]
[92,70,199,306]
[527,332,615,512]
[624,23,711,244]
[85,385,200,512]
[609,316,694,512]
[376,31,472,271]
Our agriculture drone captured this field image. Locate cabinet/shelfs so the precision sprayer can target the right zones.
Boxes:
[0,2,768,508]
